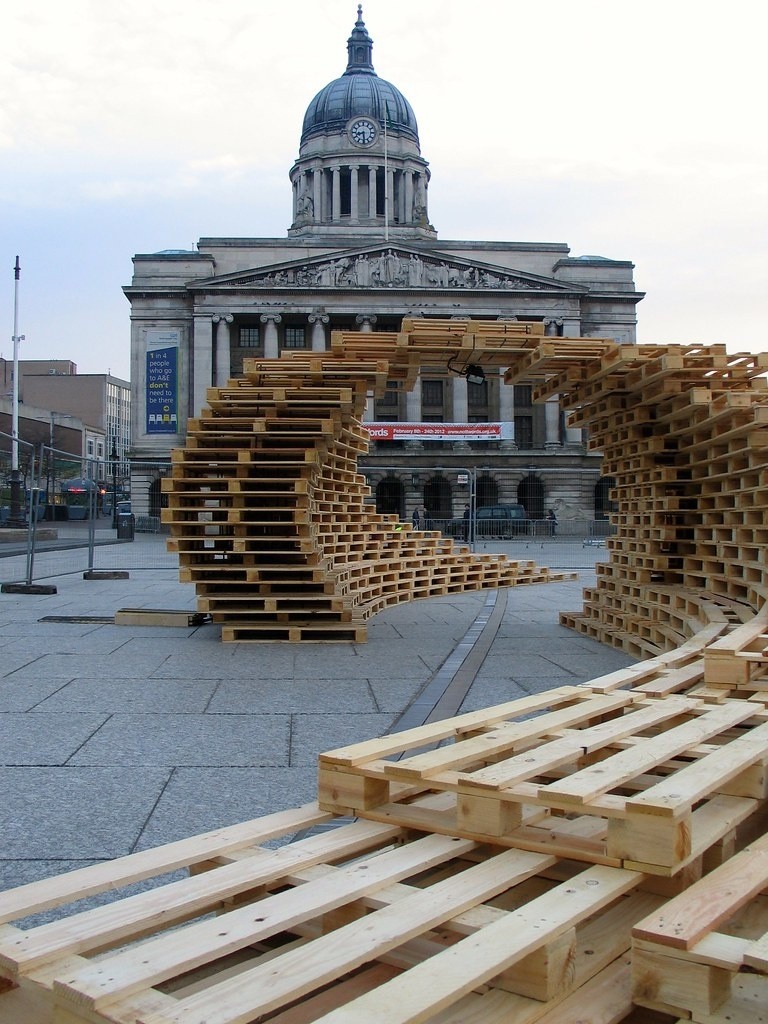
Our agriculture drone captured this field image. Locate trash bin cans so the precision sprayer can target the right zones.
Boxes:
[116,513,135,542]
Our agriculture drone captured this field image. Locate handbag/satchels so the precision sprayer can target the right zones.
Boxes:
[552,520,558,525]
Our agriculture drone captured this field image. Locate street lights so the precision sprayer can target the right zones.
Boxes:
[107,434,122,530]
[35,411,72,523]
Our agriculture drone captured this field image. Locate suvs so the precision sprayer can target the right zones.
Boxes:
[444,504,533,541]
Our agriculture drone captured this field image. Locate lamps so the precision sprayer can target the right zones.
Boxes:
[446,356,485,386]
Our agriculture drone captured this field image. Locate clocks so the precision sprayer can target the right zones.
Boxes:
[346,115,381,150]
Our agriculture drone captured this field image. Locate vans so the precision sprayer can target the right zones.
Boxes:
[115,501,132,514]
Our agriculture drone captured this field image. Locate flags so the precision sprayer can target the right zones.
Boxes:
[386,101,392,129]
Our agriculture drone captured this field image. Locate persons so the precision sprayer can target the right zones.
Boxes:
[423,506,431,531]
[274,249,474,287]
[412,507,420,530]
[464,503,474,542]
[548,508,558,538]
